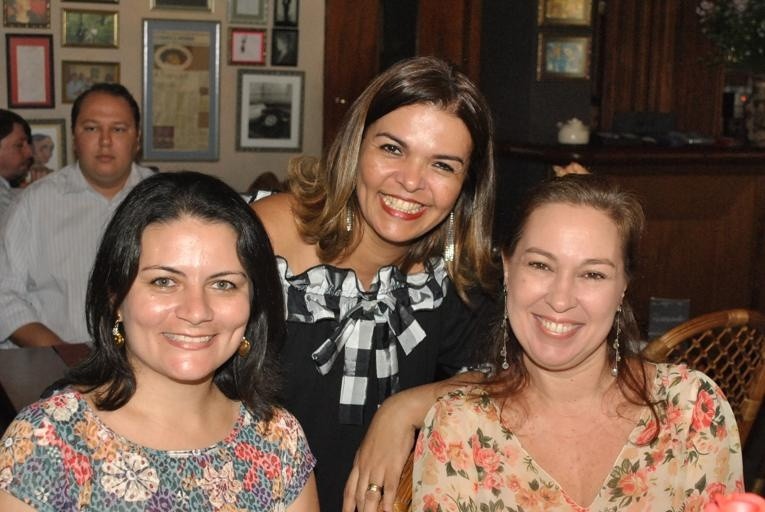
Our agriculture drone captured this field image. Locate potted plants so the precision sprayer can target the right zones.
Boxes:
[694,0,765,149]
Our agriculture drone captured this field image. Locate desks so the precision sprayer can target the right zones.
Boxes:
[488,127,765,345]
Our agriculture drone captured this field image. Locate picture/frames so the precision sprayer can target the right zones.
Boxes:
[60,6,122,49]
[138,16,221,163]
[19,116,68,190]
[6,33,58,110]
[149,0,215,14]
[59,59,124,105]
[226,28,268,66]
[2,0,53,31]
[536,29,592,85]
[234,66,307,155]
[270,26,299,67]
[273,0,301,27]
[227,0,270,29]
[535,1,595,31]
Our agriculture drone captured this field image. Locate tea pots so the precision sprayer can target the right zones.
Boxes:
[557,118,590,146]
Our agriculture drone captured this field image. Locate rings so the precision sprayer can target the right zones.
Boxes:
[367,483,382,494]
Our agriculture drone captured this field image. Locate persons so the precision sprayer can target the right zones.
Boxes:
[0,83,157,348]
[248,57,499,512]
[0,108,48,214]
[0,172,321,512]
[411,174,744,512]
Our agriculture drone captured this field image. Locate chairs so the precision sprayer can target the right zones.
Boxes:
[636,307,765,452]
